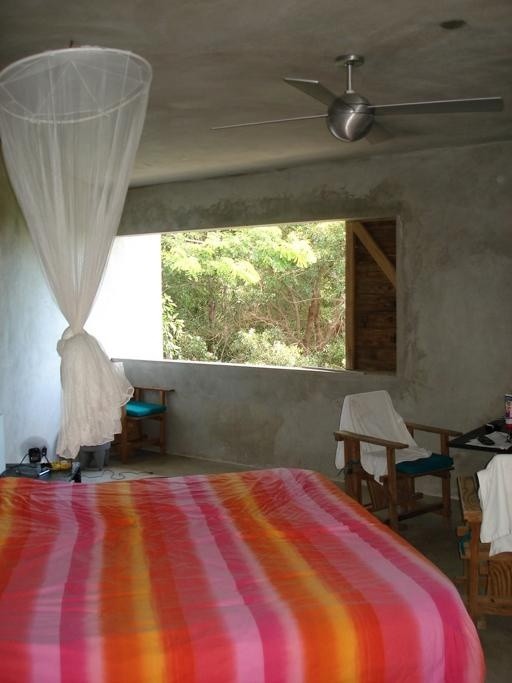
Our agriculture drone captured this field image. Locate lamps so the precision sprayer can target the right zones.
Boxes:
[17,447,42,464]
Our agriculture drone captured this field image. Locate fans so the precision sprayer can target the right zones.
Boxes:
[211,52,507,142]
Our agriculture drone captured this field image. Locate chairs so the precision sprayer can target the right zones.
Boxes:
[110,385,175,464]
[449,454,512,627]
[333,390,464,532]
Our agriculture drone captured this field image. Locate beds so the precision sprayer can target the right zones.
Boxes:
[0,467,487,683]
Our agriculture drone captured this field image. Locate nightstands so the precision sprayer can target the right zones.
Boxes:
[0,461,82,483]
